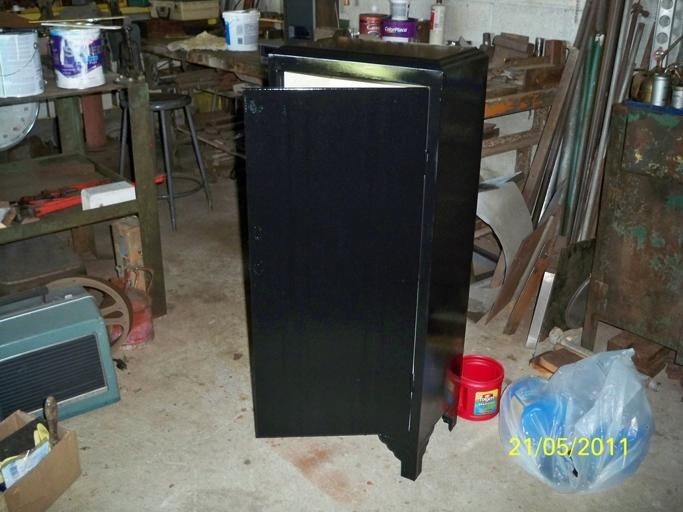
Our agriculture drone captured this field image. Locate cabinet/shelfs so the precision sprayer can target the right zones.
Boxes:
[0,70,168,318]
[244,43,488,482]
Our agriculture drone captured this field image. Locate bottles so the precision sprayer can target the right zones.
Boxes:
[428,0,447,46]
[630,50,683,111]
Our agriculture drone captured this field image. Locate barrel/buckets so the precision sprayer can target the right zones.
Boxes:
[458,355,504,421]
[0,29,45,97]
[441,358,458,417]
[222,10,260,51]
[49,20,107,90]
[502,378,651,487]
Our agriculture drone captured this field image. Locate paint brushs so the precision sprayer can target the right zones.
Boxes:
[28,16,127,30]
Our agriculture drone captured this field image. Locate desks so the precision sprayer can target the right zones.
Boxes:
[121,38,564,285]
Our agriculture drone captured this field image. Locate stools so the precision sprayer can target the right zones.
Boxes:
[119,93,214,232]
